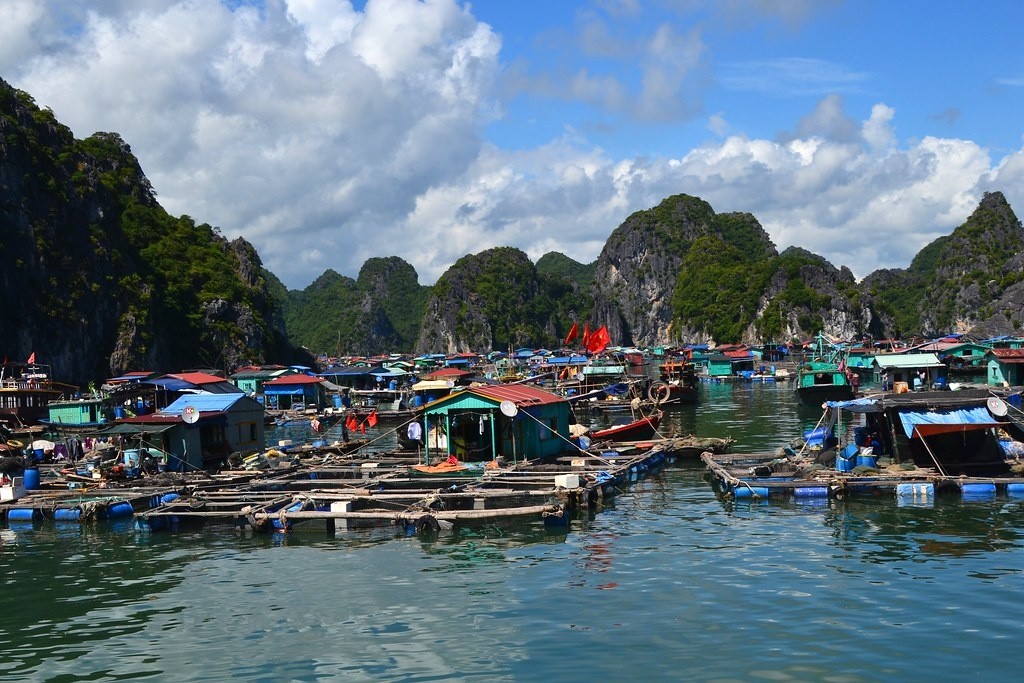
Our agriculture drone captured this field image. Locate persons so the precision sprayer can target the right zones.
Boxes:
[852,374,860,393]
[100,413,107,420]
[227,452,246,469]
[881,375,888,391]
[0,472,12,487]
[140,449,158,477]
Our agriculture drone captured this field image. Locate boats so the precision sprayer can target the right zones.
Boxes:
[0,353,81,428]
[38,389,113,434]
[656,356,700,406]
[793,330,852,408]
[0,425,48,438]
[568,398,664,452]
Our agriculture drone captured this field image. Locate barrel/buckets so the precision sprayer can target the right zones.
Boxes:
[150,493,180,507]
[731,486,768,498]
[804,425,883,472]
[24,466,40,489]
[545,452,667,527]
[7,509,35,520]
[1008,392,1021,409]
[960,483,996,493]
[937,376,946,391]
[135,504,309,529]
[124,448,139,476]
[54,508,82,520]
[34,449,44,460]
[102,448,119,462]
[794,487,827,498]
[389,377,435,408]
[115,406,124,419]
[105,503,133,518]
[771,365,776,374]
[760,364,765,374]
[1007,483,1024,492]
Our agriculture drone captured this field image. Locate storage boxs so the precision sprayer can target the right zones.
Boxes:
[279,440,293,450]
[0,484,27,501]
[554,474,579,489]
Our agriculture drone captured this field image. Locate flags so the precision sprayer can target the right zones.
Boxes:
[565,321,611,354]
[27,352,35,364]
[345,410,377,435]
